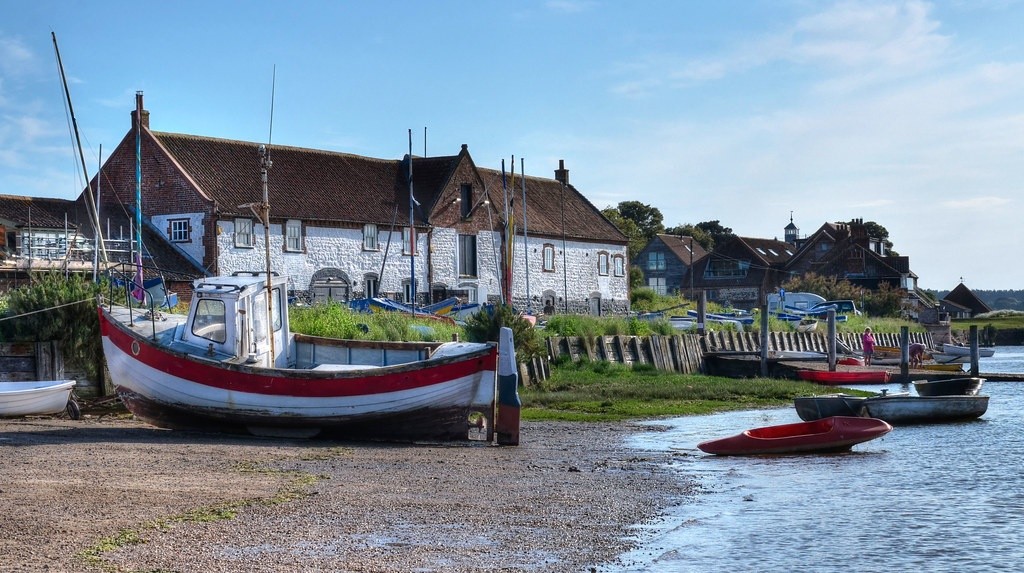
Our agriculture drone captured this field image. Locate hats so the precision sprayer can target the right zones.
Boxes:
[921,344,927,352]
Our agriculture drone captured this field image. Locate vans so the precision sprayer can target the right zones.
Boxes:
[768,292,856,317]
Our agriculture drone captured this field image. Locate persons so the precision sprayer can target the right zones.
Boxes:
[543,300,556,315]
[862,327,875,366]
[909,343,926,369]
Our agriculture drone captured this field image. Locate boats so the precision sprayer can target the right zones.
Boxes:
[839,357,868,366]
[909,363,963,371]
[697,416,893,454]
[97,64,498,438]
[794,395,990,422]
[0,380,76,416]
[944,343,995,356]
[797,370,891,384]
[914,377,987,396]
[932,354,980,363]
